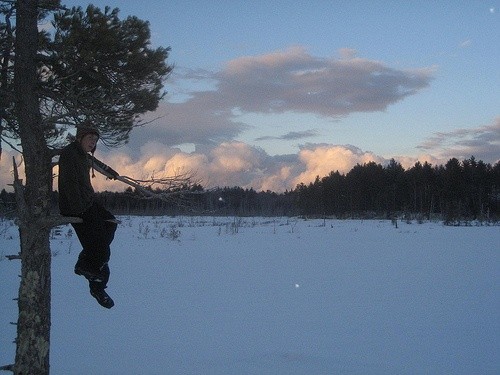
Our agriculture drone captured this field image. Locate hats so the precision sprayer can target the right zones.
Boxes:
[76,119,100,153]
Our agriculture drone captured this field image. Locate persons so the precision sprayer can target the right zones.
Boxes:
[57,120,116,309]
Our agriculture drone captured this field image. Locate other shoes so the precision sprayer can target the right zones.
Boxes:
[89,287,114,309]
[73,262,104,282]
